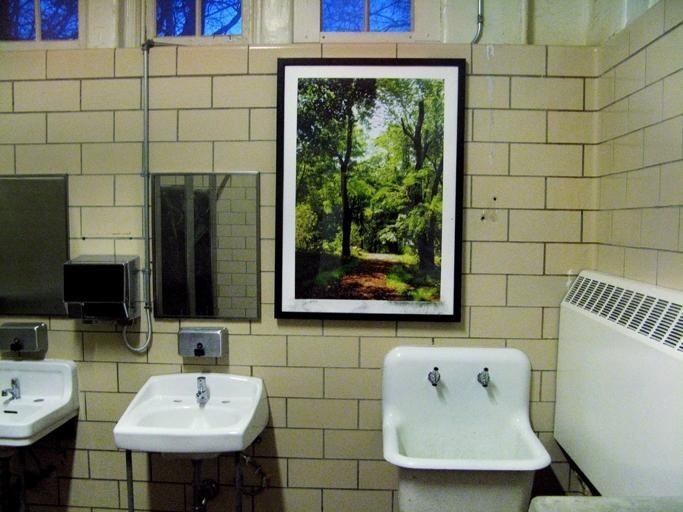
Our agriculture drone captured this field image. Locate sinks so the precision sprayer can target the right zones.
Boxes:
[0,359,79,446]
[113,373,270,455]
[382,346,551,510]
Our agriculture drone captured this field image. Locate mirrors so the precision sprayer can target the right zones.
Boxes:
[151,171,261,320]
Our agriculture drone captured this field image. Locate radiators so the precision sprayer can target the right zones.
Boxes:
[553,270,683,497]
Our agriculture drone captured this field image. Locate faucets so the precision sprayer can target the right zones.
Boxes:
[1,378,22,399]
[478,367,490,389]
[428,366,441,387]
[194,376,210,405]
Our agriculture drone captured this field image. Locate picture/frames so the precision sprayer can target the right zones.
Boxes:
[274,57,466,320]
